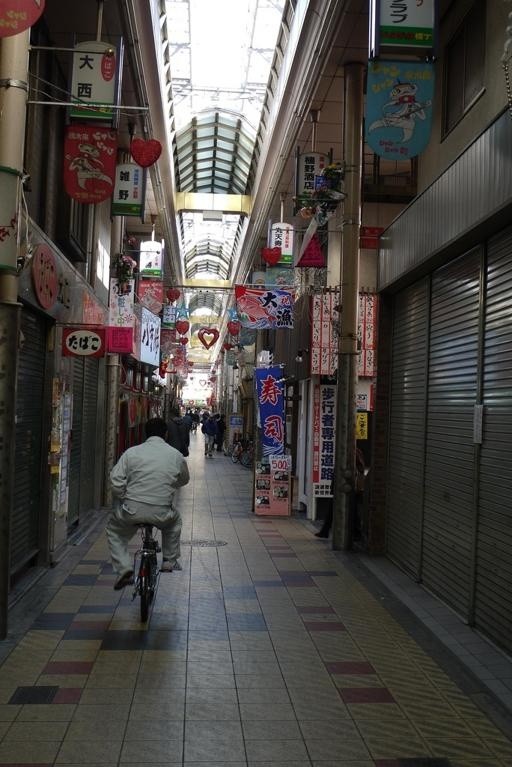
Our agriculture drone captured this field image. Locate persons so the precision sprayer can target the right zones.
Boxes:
[164,405,227,457]
[312,472,335,540]
[104,416,191,591]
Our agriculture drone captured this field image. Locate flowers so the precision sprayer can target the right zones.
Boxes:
[110,253,138,281]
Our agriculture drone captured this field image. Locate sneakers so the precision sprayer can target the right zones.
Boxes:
[114,566,134,590]
[161,559,182,571]
[314,532,329,538]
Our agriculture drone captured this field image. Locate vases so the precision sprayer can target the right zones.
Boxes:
[121,280,129,293]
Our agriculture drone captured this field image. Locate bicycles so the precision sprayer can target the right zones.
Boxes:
[230,437,255,468]
[130,521,162,624]
